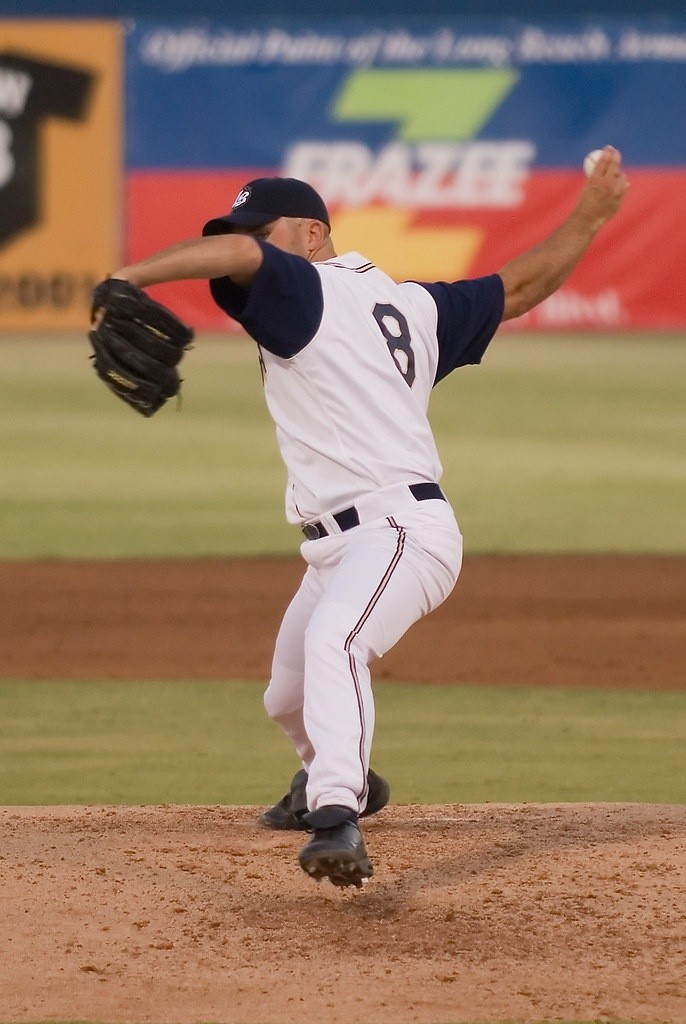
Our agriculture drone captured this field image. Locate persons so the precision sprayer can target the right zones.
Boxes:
[89,146,628,887]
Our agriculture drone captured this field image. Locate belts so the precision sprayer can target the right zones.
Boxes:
[300,483,447,540]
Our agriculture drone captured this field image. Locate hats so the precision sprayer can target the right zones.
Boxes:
[202,177,330,236]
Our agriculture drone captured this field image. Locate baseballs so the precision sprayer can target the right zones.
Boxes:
[582,149,605,177]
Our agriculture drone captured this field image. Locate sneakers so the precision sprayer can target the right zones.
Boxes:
[256,767,389,830]
[298,807,374,889]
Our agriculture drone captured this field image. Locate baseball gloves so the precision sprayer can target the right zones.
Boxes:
[87,279,195,420]
